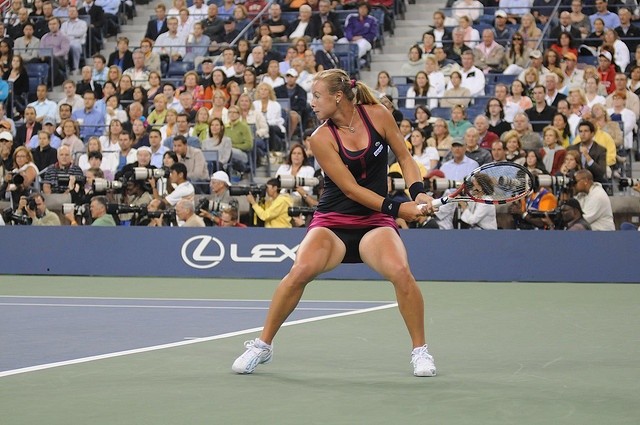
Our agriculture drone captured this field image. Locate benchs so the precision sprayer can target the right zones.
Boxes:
[0,0,412,228]
[2,2,134,132]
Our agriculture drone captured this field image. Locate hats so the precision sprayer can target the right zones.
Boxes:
[452,137,466,147]
[0,131,13,141]
[286,69,298,77]
[495,10,506,18]
[563,53,577,62]
[560,198,584,213]
[599,50,612,61]
[529,50,542,59]
[210,171,232,186]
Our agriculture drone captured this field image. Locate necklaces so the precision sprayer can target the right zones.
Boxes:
[333,108,358,133]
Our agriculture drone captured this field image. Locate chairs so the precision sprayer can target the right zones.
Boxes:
[412,0,636,70]
[611,121,632,181]
[384,72,519,126]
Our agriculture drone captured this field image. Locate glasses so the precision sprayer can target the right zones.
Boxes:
[512,36,523,41]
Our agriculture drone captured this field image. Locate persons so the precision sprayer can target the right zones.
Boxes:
[232,69,436,377]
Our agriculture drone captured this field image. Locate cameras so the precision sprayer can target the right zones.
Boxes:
[391,178,431,192]
[433,177,473,196]
[92,178,129,193]
[535,170,577,189]
[1,206,32,225]
[498,176,526,192]
[209,199,239,213]
[132,166,170,181]
[287,206,317,217]
[194,197,209,215]
[229,183,266,197]
[618,178,640,187]
[57,174,86,186]
[531,207,562,223]
[105,202,148,215]
[148,209,176,222]
[22,198,37,215]
[277,175,324,189]
[61,203,89,217]
[7,172,24,185]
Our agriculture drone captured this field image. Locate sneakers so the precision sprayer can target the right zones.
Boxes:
[231,336,273,374]
[410,343,437,377]
